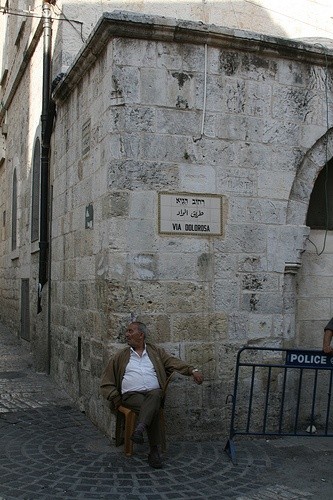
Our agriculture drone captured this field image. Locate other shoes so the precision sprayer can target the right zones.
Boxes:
[129,428,145,445]
[147,451,163,469]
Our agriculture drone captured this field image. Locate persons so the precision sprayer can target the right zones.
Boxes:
[323,317,333,353]
[99,321,204,469]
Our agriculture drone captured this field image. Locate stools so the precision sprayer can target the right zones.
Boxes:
[116,406,165,457]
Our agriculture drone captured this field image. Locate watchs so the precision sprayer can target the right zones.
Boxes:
[192,369,200,373]
